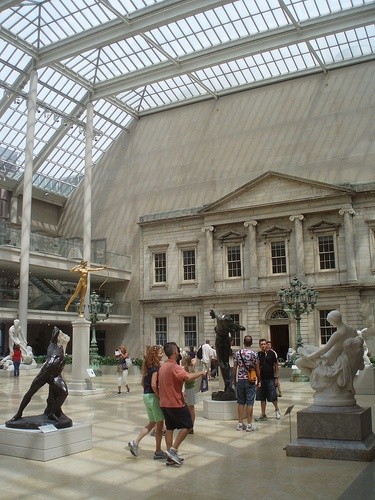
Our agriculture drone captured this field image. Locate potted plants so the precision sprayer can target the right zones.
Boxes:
[128,357,143,375]
[100,357,120,374]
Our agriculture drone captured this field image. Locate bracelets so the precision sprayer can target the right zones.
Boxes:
[274,376,278,379]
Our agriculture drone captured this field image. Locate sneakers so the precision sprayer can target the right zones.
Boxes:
[254,415,267,421]
[246,425,255,432]
[236,422,246,431]
[165,448,182,464]
[154,450,167,459]
[275,408,280,419]
[127,440,138,457]
[166,458,184,465]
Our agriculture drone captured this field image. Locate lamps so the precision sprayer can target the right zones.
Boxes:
[36,105,40,113]
[4,88,23,104]
[46,108,73,129]
[79,126,98,141]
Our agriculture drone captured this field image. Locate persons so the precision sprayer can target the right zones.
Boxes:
[254,339,282,421]
[157,342,207,466]
[11,325,70,422]
[286,348,296,363]
[11,343,24,377]
[128,345,166,460]
[0,319,34,364]
[232,335,261,433]
[115,345,131,394]
[150,338,220,437]
[227,337,236,393]
[65,259,104,316]
[209,307,246,395]
[294,310,360,398]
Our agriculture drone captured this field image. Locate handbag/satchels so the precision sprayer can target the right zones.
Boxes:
[197,346,202,359]
[248,366,256,383]
[125,356,132,368]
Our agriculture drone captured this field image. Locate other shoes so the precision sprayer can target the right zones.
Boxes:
[151,429,167,436]
